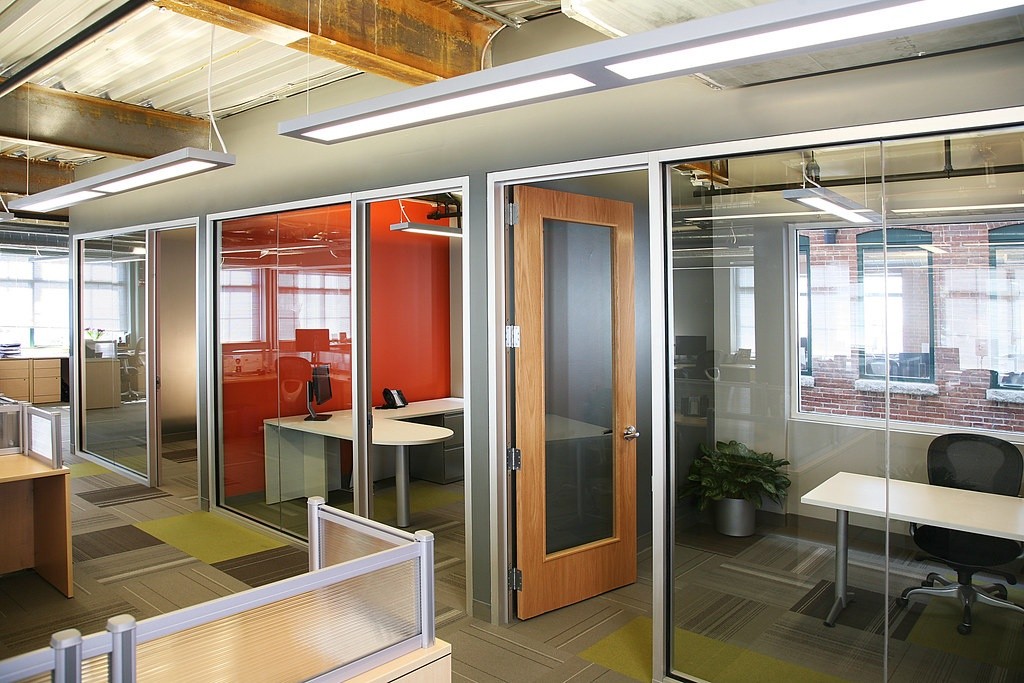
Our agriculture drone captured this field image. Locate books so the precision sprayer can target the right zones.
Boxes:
[0,343,22,358]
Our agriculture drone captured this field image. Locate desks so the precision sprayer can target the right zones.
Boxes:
[263,398,464,505]
[801,472,1024,627]
[0,396,73,598]
[224,369,352,431]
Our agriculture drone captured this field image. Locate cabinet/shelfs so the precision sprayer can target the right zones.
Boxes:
[86,359,120,410]
[0,359,63,404]
[409,411,463,484]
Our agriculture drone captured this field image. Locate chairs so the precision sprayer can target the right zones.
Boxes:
[274,357,336,416]
[896,434,1024,634]
[117,338,145,400]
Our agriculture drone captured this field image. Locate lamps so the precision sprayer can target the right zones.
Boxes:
[277,0,1024,147]
[389,222,462,238]
[782,185,885,225]
[8,148,235,214]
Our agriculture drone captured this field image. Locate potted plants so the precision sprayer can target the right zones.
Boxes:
[687,440,791,537]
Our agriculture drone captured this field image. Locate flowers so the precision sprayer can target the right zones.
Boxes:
[85,326,105,340]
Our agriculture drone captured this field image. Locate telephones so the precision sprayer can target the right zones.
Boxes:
[382,388,409,409]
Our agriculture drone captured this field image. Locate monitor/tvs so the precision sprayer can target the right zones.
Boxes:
[303,364,332,421]
[296,328,332,365]
